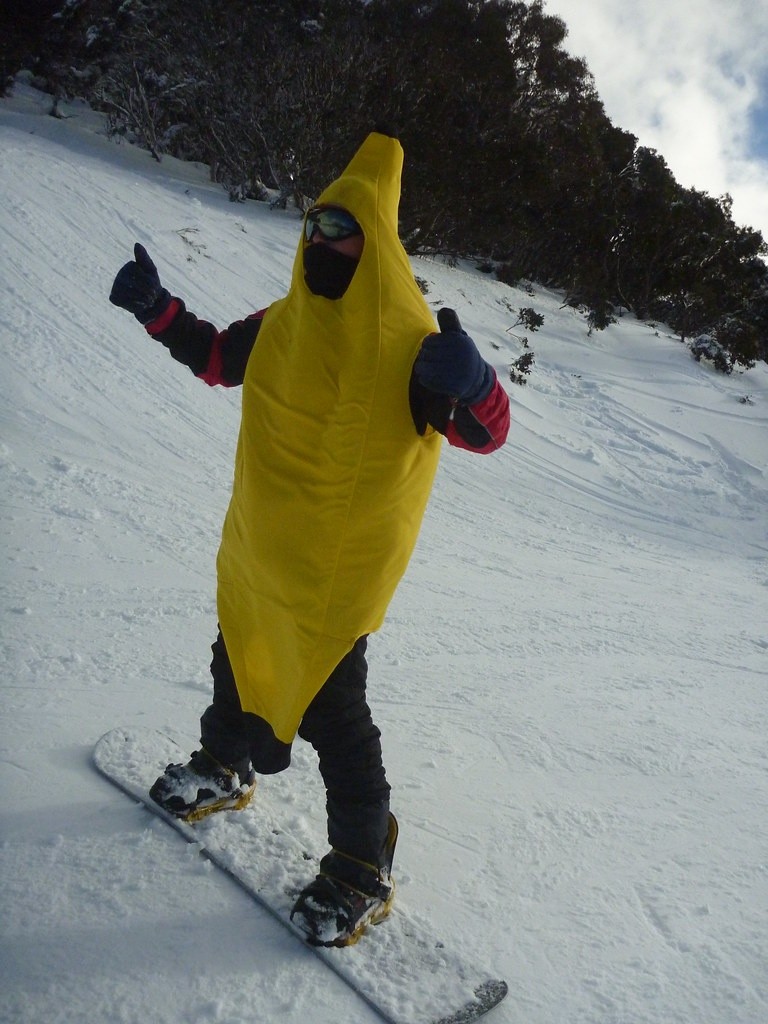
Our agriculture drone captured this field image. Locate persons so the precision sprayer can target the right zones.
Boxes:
[106,170,515,952]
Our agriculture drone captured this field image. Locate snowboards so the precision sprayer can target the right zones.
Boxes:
[87,722,510,1024]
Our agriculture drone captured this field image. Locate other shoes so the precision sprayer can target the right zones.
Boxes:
[149,747,249,812]
[291,857,386,937]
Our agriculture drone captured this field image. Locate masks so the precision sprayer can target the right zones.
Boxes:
[302,243,357,300]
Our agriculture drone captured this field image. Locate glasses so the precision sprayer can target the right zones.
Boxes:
[305,208,361,241]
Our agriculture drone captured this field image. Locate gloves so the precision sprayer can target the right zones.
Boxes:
[109,242,171,323]
[413,308,496,409]
